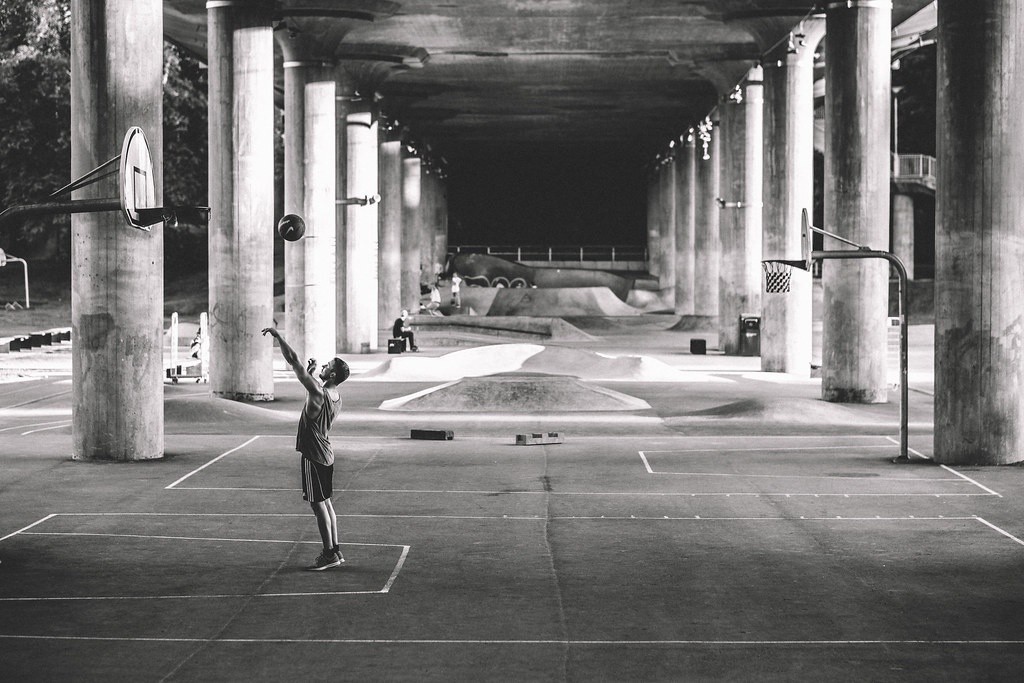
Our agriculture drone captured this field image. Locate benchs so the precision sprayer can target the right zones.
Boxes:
[388,338,406,354]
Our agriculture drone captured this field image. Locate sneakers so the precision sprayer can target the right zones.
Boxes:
[309,551,340,571]
[337,551,344,564]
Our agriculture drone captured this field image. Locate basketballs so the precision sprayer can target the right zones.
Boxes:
[277,214,306,242]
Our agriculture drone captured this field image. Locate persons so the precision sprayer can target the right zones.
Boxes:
[450,272,463,309]
[261,326,350,571]
[393,310,418,350]
[426,283,442,316]
[469,279,538,288]
